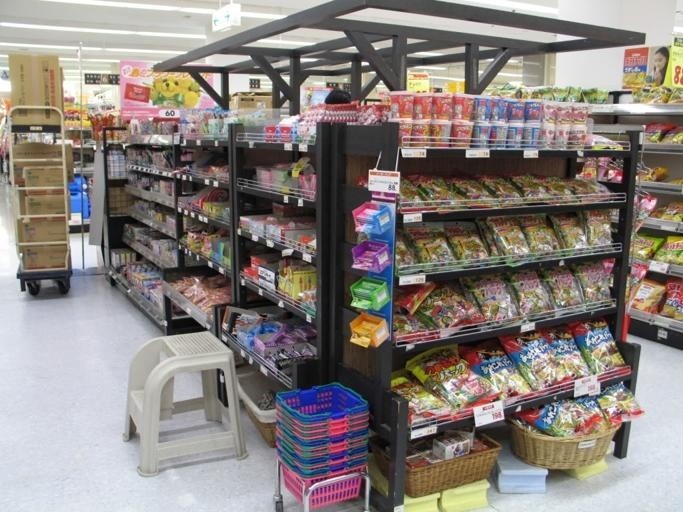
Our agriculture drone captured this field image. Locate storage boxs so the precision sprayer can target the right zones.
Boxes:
[5,52,65,130]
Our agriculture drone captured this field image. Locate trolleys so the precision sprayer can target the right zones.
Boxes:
[272,457,371,511]
[6,105,73,295]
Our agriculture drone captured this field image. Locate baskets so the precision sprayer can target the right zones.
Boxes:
[274,383,369,508]
[506,421,623,470]
[371,427,502,498]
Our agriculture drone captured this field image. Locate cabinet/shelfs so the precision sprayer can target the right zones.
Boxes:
[0,92,683,512]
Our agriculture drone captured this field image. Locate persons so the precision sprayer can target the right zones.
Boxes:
[324,88,352,105]
[650,47,670,87]
